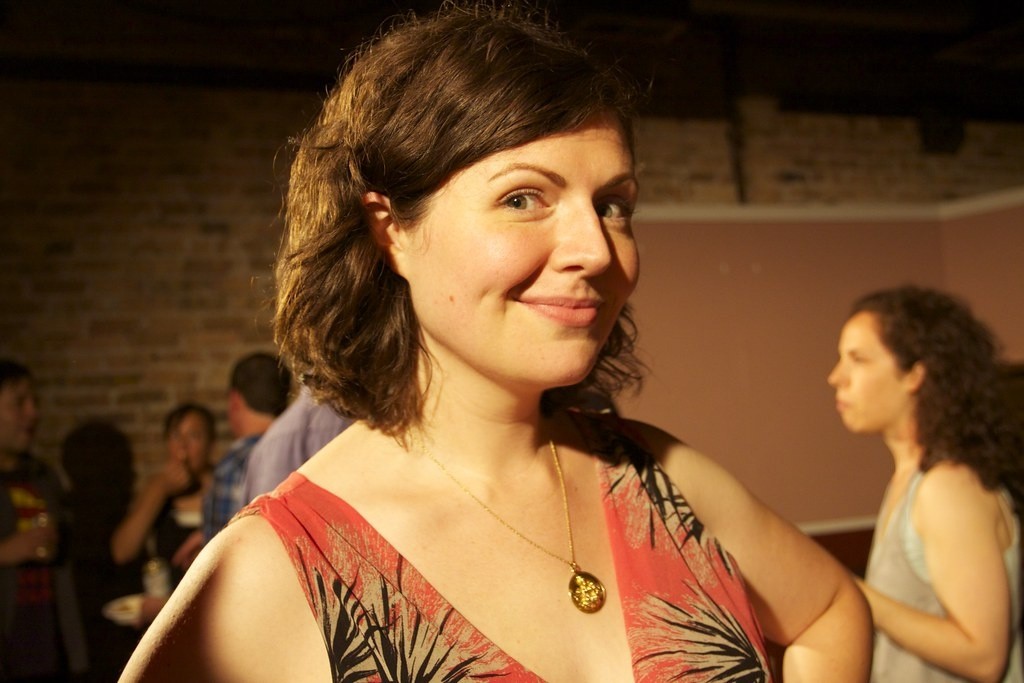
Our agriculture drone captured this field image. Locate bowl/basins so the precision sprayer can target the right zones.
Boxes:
[104,594,144,624]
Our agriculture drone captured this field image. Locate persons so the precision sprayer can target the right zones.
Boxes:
[828,282,1024,683]
[0,0,873,683]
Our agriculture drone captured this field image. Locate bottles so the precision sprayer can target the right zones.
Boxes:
[145,561,171,599]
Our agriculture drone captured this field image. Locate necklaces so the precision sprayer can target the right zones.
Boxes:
[403,415,606,614]
